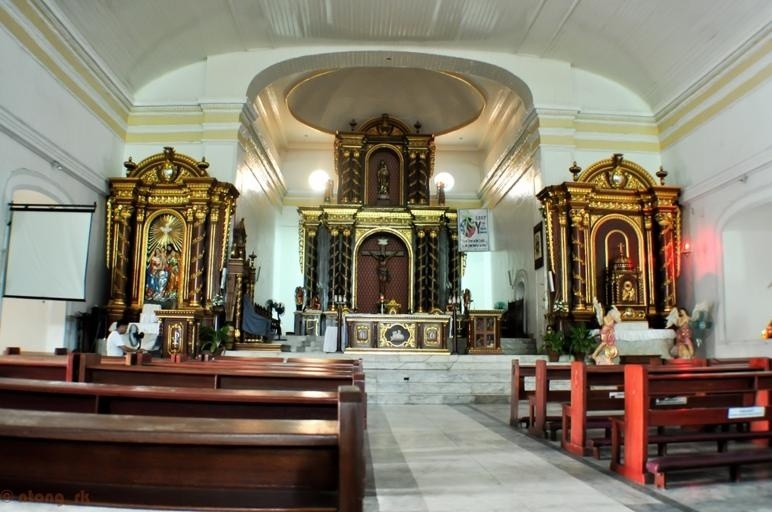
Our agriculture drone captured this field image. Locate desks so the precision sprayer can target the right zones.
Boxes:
[344,314,453,356]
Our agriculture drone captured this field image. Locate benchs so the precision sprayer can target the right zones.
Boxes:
[1,342,369,511]
[508,354,771,492]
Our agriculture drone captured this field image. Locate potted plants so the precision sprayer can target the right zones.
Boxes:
[536,325,567,362]
[561,319,601,361]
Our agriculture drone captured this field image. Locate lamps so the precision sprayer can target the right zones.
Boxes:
[307,165,336,207]
[433,169,457,206]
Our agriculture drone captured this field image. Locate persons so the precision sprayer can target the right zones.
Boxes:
[588,313,618,359]
[106,319,140,356]
[369,251,398,299]
[148,244,181,298]
[675,307,693,357]
[377,159,390,195]
